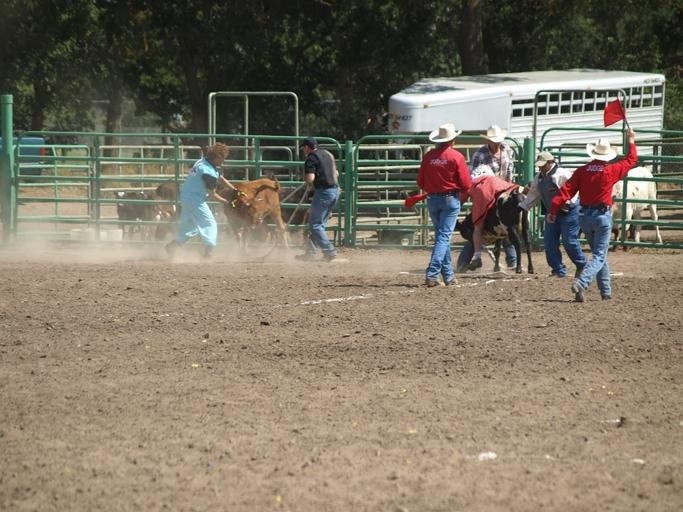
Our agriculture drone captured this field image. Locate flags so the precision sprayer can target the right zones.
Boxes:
[405,193,426,209]
[604,100,626,128]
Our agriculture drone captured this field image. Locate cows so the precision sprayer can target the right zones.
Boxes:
[114,191,159,241]
[156,182,178,222]
[264,187,315,248]
[612,167,663,252]
[217,175,289,253]
[454,186,534,273]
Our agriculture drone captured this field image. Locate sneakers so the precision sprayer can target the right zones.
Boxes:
[468,259,482,270]
[294,253,315,262]
[319,256,338,263]
[601,294,611,300]
[571,282,585,301]
[428,278,458,286]
[549,270,565,278]
[575,267,584,277]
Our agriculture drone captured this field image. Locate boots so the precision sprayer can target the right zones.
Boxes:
[204,245,216,263]
[165,240,182,256]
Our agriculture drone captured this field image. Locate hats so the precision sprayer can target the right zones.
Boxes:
[586,139,618,162]
[299,137,316,148]
[479,125,508,143]
[428,123,463,143]
[534,151,554,167]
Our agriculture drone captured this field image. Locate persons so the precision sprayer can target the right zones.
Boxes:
[517,150,587,279]
[161,140,246,264]
[457,123,519,273]
[461,164,525,270]
[414,123,470,287]
[292,138,340,263]
[545,126,639,303]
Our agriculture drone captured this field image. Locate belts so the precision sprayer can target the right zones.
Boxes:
[315,184,338,190]
[580,205,598,209]
[428,191,457,197]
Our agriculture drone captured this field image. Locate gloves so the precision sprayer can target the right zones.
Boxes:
[225,189,248,208]
